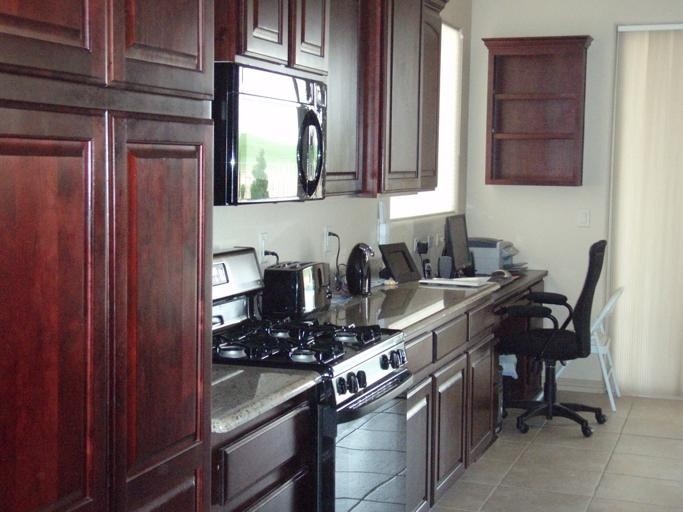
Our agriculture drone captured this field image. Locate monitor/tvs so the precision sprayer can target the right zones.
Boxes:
[437,213,472,277]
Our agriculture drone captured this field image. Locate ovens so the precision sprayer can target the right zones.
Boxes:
[320,372,407,512]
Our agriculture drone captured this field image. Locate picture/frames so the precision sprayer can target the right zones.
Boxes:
[379,242,422,284]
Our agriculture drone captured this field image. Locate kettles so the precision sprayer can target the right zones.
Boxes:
[344,243,373,295]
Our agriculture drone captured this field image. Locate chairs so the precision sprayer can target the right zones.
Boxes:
[499,239,608,437]
[536,287,625,412]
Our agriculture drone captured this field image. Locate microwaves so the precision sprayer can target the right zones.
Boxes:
[214,60,327,205]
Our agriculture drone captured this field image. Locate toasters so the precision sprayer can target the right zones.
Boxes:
[261,259,331,316]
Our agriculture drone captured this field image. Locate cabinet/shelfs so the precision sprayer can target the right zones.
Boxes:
[214,0,331,86]
[504,280,544,401]
[405,298,498,512]
[481,35,594,187]
[0,0,214,512]
[323,0,381,198]
[211,388,319,512]
[378,0,451,195]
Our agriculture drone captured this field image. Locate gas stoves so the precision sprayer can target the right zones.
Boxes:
[212,315,408,405]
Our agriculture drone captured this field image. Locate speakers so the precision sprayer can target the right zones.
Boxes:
[438,255,455,278]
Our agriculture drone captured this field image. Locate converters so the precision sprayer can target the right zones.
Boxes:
[417,241,428,254]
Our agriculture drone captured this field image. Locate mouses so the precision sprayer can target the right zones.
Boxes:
[493,270,512,278]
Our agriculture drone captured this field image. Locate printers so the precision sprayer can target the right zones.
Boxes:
[468,237,528,275]
